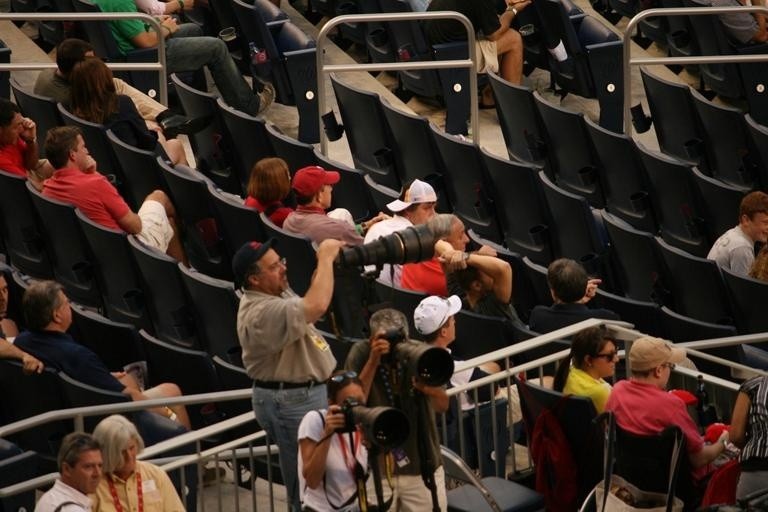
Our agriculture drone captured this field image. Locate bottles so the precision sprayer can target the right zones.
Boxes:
[248,41,259,65]
[398,48,411,62]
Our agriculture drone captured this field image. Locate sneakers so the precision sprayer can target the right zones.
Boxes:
[198,467,226,487]
[257,82,275,113]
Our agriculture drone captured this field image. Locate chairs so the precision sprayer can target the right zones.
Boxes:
[0,0,768,512]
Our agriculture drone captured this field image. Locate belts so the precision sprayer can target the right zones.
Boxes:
[255,380,327,389]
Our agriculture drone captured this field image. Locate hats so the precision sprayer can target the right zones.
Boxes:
[291,165,341,199]
[386,179,437,212]
[629,338,686,371]
[414,295,462,335]
[232,236,277,279]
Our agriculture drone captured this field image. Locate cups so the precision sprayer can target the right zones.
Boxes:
[219,25,240,53]
[520,24,535,36]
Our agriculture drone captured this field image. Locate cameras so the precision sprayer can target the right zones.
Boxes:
[341,223,436,269]
[332,396,412,449]
[378,326,455,398]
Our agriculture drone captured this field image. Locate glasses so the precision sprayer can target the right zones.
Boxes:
[330,371,357,383]
[591,350,617,361]
[257,257,287,277]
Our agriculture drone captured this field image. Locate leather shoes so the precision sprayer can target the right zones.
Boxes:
[159,114,212,138]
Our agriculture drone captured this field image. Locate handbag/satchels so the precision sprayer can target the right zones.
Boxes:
[596,474,683,512]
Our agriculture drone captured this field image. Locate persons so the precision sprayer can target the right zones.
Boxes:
[0,274,193,432]
[296,374,368,512]
[729,374,768,507]
[34,38,191,170]
[1,100,199,273]
[413,295,554,426]
[705,191,767,279]
[247,158,618,333]
[231,237,346,511]
[35,431,104,511]
[94,0,277,118]
[345,310,450,510]
[709,2,766,45]
[409,1,533,109]
[605,335,730,493]
[85,415,186,511]
[551,327,619,416]
